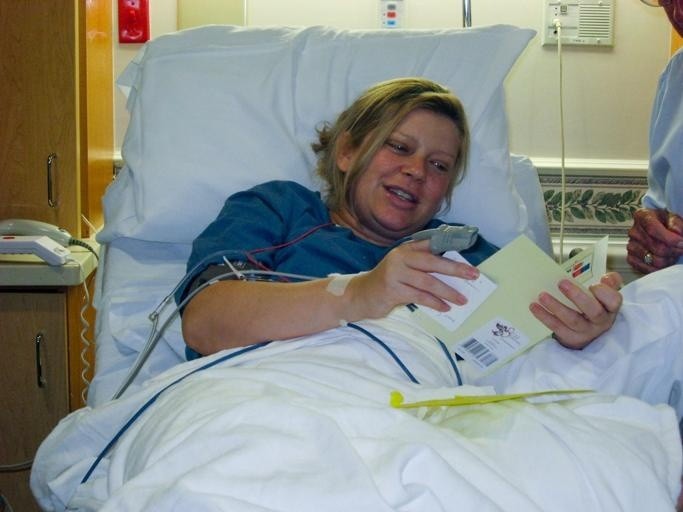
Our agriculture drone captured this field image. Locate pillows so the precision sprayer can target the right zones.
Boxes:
[93,25,539,251]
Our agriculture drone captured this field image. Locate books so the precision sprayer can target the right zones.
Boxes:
[393,234,609,376]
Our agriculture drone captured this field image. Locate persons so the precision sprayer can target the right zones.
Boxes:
[626,0,683,276]
[173,77,622,361]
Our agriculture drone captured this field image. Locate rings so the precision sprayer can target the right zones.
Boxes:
[643,252,653,266]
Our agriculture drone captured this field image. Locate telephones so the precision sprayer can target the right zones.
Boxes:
[0,235,71,266]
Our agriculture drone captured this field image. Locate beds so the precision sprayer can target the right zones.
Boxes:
[88,25,559,511]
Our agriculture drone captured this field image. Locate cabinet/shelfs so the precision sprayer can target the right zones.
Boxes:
[0,0,113,241]
[0,285,72,512]
